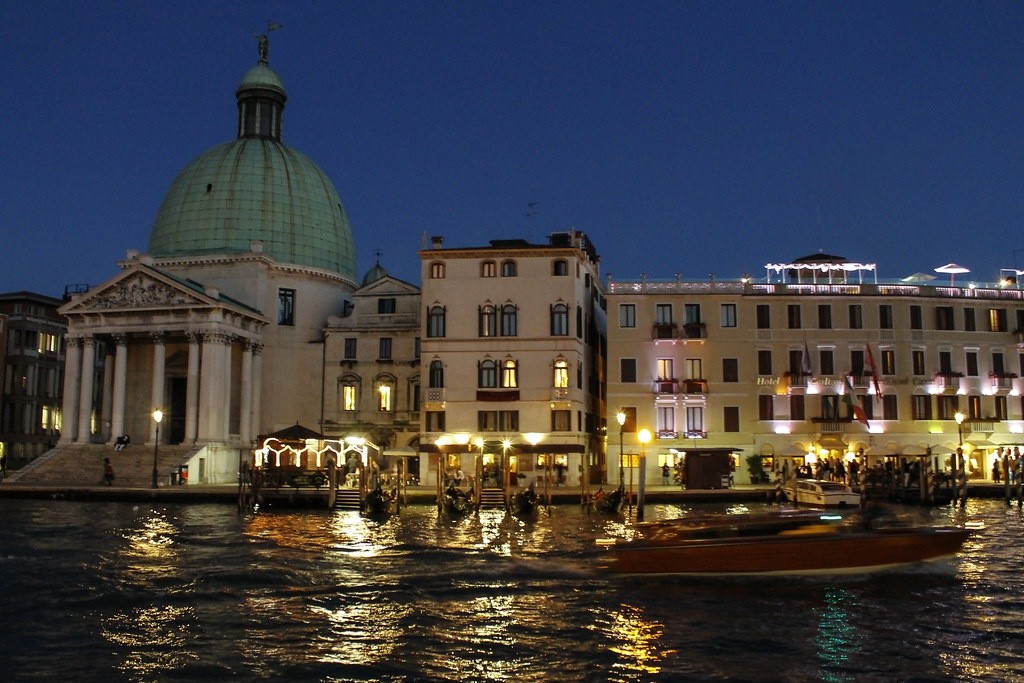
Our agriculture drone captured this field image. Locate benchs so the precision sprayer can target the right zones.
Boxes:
[545,475,568,488]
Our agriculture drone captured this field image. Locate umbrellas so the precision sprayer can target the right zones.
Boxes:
[261,420,328,449]
[934,263,971,287]
[901,272,937,282]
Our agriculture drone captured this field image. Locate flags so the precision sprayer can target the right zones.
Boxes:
[865,342,882,403]
[802,337,813,373]
[844,377,870,428]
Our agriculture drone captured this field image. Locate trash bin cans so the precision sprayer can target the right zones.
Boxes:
[390,475,399,487]
[171,472,181,485]
[536,476,543,488]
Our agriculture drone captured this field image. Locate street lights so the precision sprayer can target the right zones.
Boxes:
[616,412,626,491]
[151,407,163,489]
[955,412,966,480]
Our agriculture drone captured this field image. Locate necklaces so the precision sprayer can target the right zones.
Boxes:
[103,458,115,485]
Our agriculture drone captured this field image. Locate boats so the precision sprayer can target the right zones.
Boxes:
[784,479,861,509]
[574,515,988,577]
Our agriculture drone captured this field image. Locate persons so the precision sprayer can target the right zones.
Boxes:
[663,463,670,485]
[454,468,461,486]
[814,458,864,485]
[993,459,1000,483]
[801,462,812,478]
[114,435,130,451]
[344,453,360,472]
[1002,454,1014,484]
[782,459,788,481]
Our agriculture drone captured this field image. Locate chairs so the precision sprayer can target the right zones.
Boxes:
[347,467,360,487]
